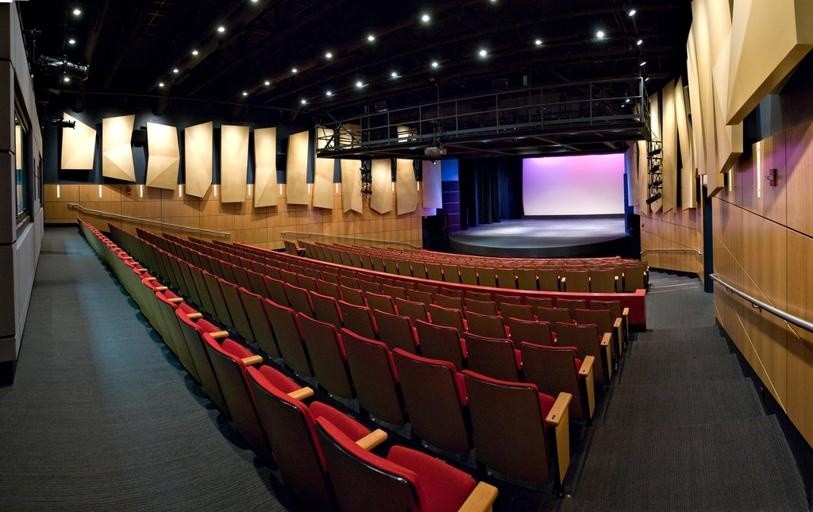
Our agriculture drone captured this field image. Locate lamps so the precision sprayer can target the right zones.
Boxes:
[646,193,662,205]
[647,164,661,175]
[647,148,661,158]
[649,180,663,190]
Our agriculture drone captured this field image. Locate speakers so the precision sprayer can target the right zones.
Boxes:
[131,129,147,147]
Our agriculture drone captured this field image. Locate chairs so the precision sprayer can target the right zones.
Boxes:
[75,216,651,510]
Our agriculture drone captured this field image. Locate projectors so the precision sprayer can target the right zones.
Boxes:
[425,146,447,158]
